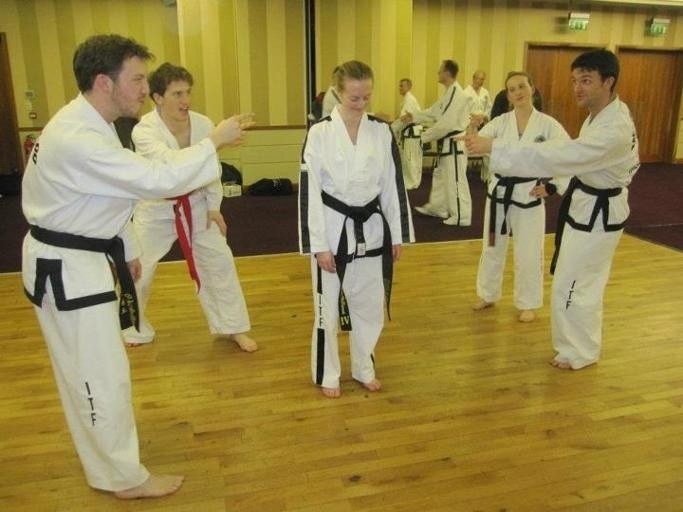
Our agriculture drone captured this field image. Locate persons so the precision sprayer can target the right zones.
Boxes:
[18,34,256,500]
[119,62,256,354]
[450,48,640,371]
[469,73,580,325]
[401,60,473,227]
[320,65,391,120]
[490,79,541,121]
[462,71,486,181]
[390,78,423,191]
[296,60,415,399]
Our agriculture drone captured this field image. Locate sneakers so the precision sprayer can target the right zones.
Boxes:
[443,218,471,226]
[415,206,449,218]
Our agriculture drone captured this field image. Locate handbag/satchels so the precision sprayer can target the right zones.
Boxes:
[249,178,292,195]
[221,162,242,184]
